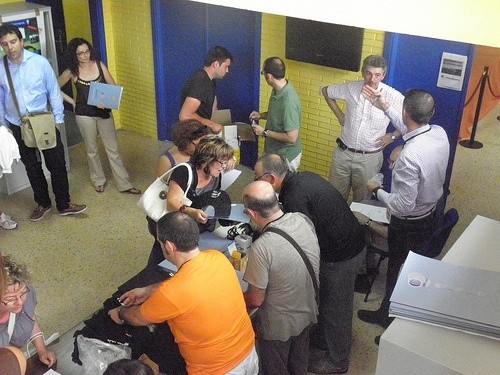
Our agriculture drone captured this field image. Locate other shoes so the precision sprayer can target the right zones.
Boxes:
[95,185,104,192]
[307,335,349,375]
[357,309,392,329]
[123,187,141,194]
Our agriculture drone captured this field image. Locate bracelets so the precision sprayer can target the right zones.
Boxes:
[372,187,380,197]
[233,155,236,161]
[180,205,188,212]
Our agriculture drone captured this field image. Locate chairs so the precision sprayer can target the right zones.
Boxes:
[364,208,459,302]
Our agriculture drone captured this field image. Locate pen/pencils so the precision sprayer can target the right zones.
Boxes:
[370,88,384,108]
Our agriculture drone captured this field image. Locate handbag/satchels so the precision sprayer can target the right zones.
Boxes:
[20,111,60,151]
[136,162,193,222]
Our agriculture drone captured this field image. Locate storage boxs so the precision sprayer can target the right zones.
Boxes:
[209,109,256,166]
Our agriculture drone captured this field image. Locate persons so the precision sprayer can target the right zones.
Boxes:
[147,138,234,264]
[254,153,366,375]
[249,57,302,171]
[57,37,141,194]
[322,55,408,204]
[0,23,87,222]
[0,255,57,367]
[103,359,154,375]
[108,212,259,375]
[179,46,233,133]
[156,118,235,178]
[241,180,320,375]
[358,85,450,346]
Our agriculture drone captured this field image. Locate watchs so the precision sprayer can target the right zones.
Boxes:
[391,135,396,140]
[263,129,267,136]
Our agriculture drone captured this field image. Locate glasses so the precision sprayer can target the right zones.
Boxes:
[254,172,271,182]
[214,159,227,167]
[261,70,267,76]
[1,286,31,306]
[76,49,91,56]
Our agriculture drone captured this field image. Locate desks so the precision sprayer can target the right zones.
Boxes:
[159,204,251,290]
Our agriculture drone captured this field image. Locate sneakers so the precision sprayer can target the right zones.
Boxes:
[0,210,19,230]
[28,204,53,222]
[58,203,87,216]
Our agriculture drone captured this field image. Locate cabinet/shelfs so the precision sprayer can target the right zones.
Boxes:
[376,215,500,375]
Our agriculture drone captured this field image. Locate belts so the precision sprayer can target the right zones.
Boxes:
[348,147,382,154]
[390,202,437,220]
